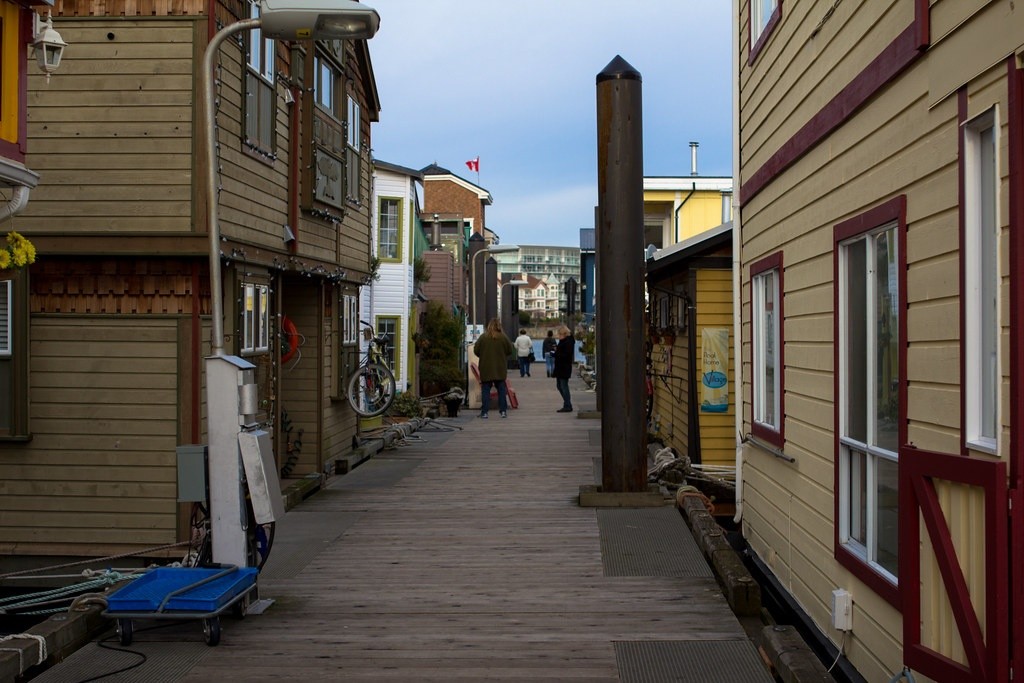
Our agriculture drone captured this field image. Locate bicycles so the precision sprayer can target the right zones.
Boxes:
[346,320,396,418]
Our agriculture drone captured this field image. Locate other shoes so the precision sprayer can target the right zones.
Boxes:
[521,373,524,377]
[481,412,488,418]
[501,412,507,417]
[557,408,571,412]
[526,372,530,376]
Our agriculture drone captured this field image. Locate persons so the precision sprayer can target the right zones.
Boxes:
[543,331,557,377]
[474,318,513,417]
[550,325,575,412]
[514,329,533,377]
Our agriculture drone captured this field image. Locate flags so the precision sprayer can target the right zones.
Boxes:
[466,158,478,172]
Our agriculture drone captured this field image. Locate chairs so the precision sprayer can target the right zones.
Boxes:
[469,361,519,409]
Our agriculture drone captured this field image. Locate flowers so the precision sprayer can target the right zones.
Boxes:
[0,230,36,268]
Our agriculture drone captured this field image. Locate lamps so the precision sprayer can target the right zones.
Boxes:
[283,225,295,244]
[27,7,70,84]
[370,259,381,272]
[284,88,295,105]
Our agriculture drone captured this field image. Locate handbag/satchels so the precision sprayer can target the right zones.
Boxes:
[528,348,535,362]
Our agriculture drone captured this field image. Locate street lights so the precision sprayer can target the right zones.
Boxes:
[196,0,382,625]
[467,245,521,411]
[499,279,529,327]
